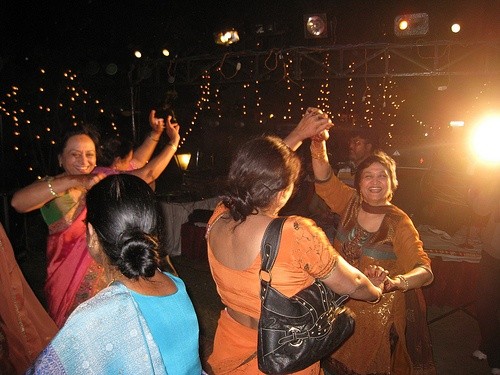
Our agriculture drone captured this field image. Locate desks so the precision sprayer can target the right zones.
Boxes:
[160,189,221,257]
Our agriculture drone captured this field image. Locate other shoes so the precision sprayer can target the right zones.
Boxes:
[492,367,500,375]
[471,349,498,364]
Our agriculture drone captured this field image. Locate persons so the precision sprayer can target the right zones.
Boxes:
[9,115,180,329]
[97,108,189,276]
[279,127,376,247]
[24,173,208,375]
[204,107,390,375]
[468,139,500,374]
[304,106,434,375]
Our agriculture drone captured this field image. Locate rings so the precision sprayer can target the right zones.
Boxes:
[375,273,379,277]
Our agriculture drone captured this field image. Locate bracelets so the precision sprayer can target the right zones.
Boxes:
[148,131,160,143]
[366,286,381,305]
[310,144,327,159]
[145,162,156,181]
[393,275,408,292]
[47,176,67,197]
[168,143,177,153]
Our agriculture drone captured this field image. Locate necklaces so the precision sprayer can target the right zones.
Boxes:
[107,264,162,288]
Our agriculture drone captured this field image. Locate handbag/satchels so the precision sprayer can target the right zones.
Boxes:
[257,217,357,375]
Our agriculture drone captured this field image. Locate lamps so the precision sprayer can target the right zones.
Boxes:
[304,12,331,39]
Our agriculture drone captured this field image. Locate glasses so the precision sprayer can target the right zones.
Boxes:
[346,140,370,145]
